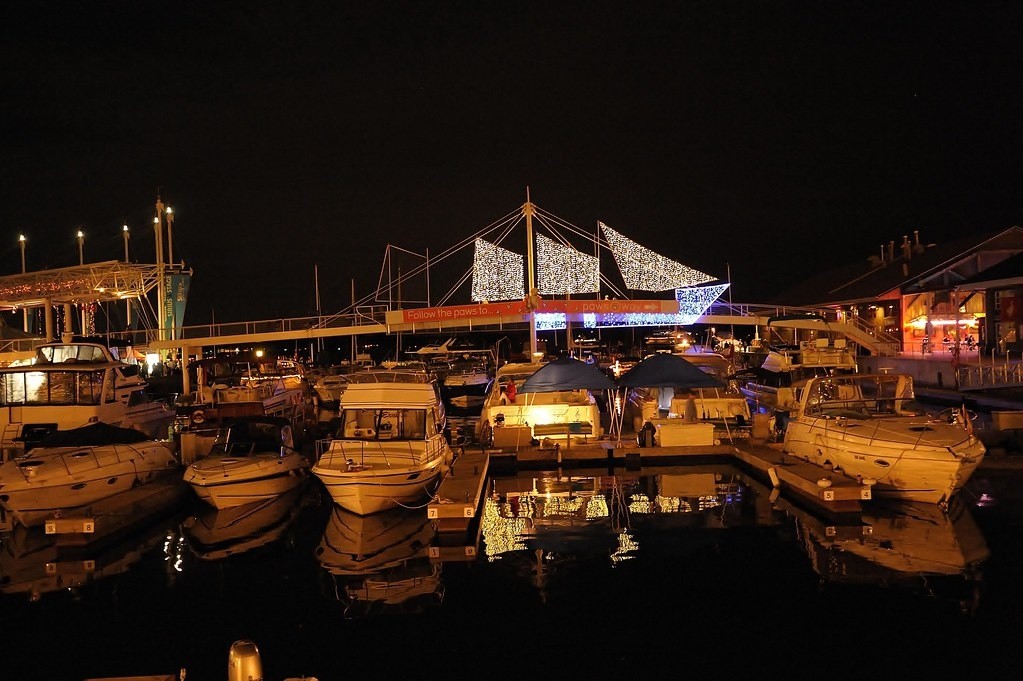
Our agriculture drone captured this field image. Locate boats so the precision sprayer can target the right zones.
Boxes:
[778,492,991,580]
[179,477,323,562]
[0,332,177,464]
[0,307,1023,517]
[0,472,185,605]
[314,503,439,579]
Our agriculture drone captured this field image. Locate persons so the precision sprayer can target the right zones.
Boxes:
[502,376,517,406]
[684,392,697,424]
[942,334,976,352]
[658,387,675,419]
[125,324,134,345]
[299,356,312,364]
[711,338,730,350]
[737,339,748,353]
[385,421,393,430]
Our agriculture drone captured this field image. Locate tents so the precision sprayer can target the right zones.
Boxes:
[609,355,733,448]
[515,358,622,452]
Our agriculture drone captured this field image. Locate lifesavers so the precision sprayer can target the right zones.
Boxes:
[192,409,204,424]
